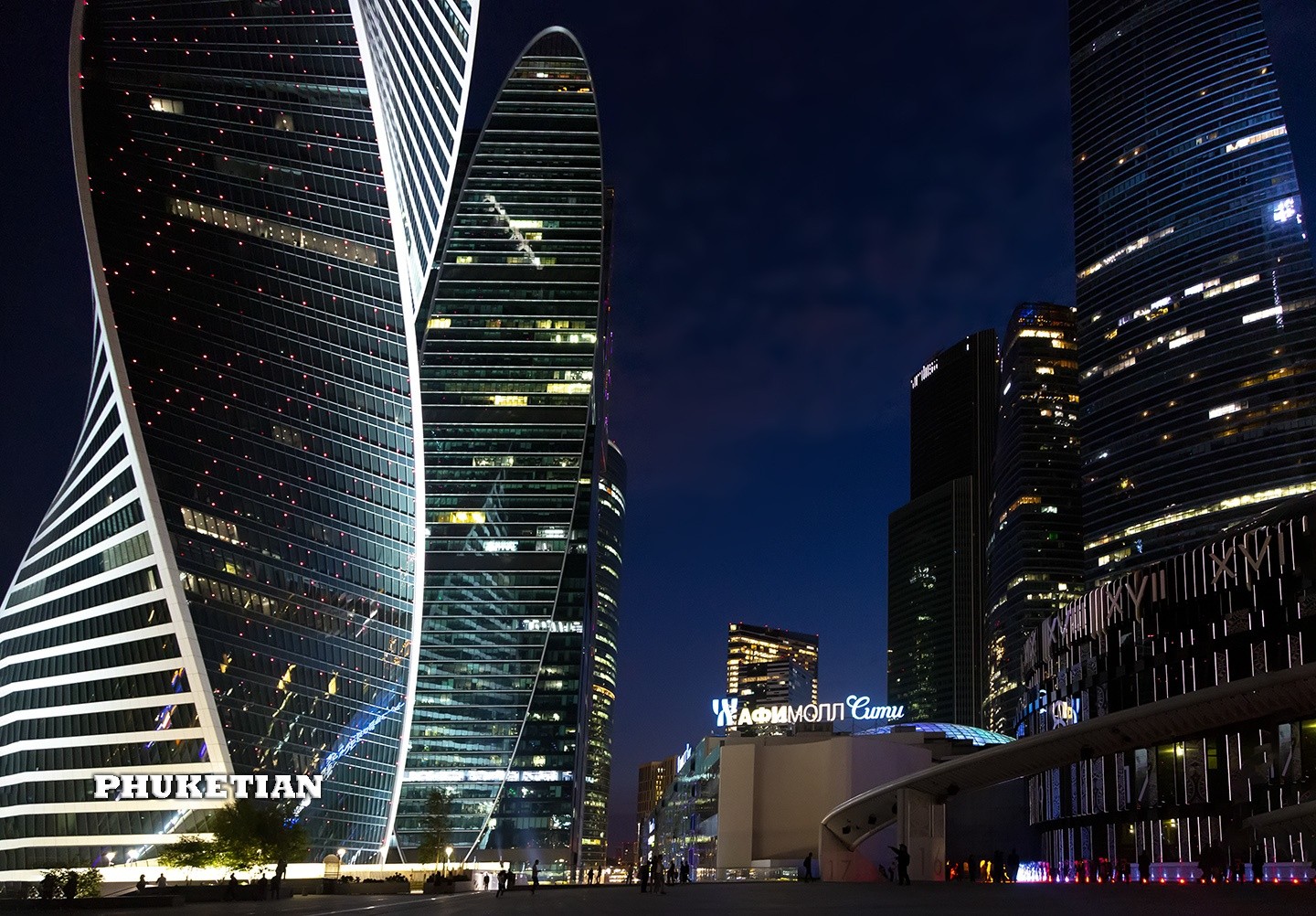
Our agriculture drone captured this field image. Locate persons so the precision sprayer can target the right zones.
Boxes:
[156,873,166,886]
[1193,842,1227,884]
[1308,860,1316,881]
[990,848,1020,884]
[587,867,594,885]
[137,875,146,892]
[879,843,910,886]
[1137,850,1152,885]
[496,868,514,899]
[596,865,602,884]
[1229,858,1245,884]
[637,854,668,894]
[1251,850,1265,881]
[1062,857,1131,885]
[666,860,692,884]
[532,859,542,895]
[257,871,280,902]
[625,864,634,884]
[803,852,813,883]
[483,873,489,890]
